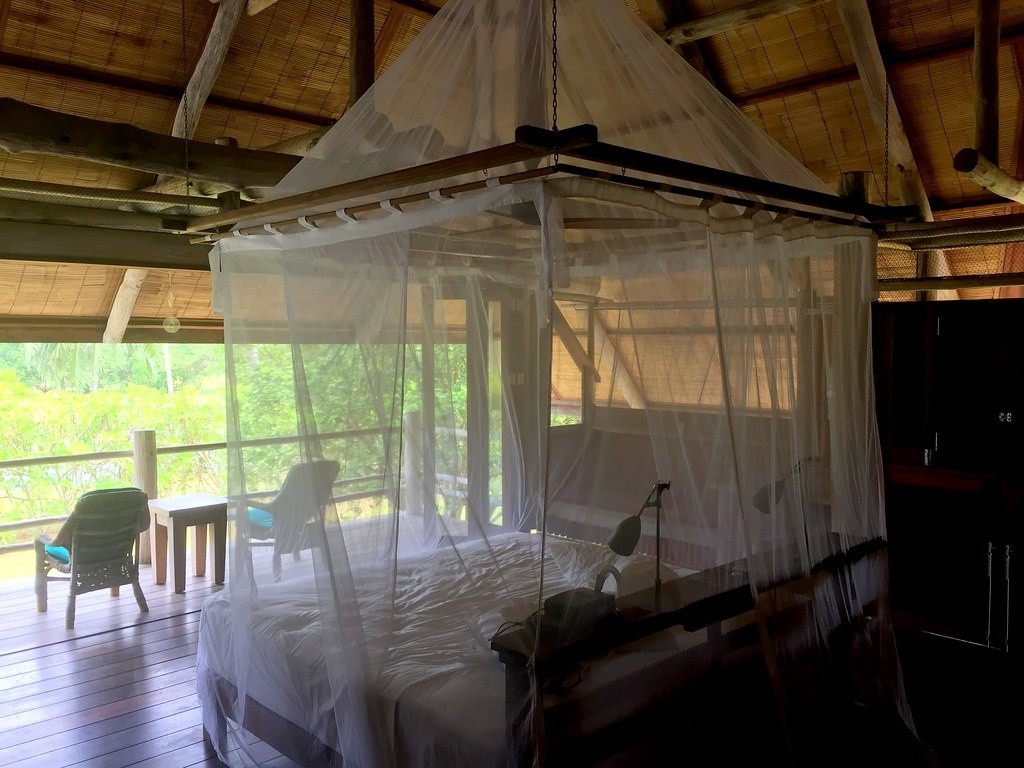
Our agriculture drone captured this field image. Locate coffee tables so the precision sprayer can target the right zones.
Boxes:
[143,493,227,593]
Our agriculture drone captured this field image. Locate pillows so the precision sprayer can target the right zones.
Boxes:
[541,541,684,596]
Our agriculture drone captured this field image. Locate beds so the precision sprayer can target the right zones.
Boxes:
[200,531,706,767]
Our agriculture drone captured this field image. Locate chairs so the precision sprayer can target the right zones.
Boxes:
[838,535,940,768]
[235,457,342,579]
[687,615,801,768]
[752,572,830,737]
[32,488,154,629]
[811,561,875,744]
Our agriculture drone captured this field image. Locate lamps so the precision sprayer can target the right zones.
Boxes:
[608,481,670,613]
